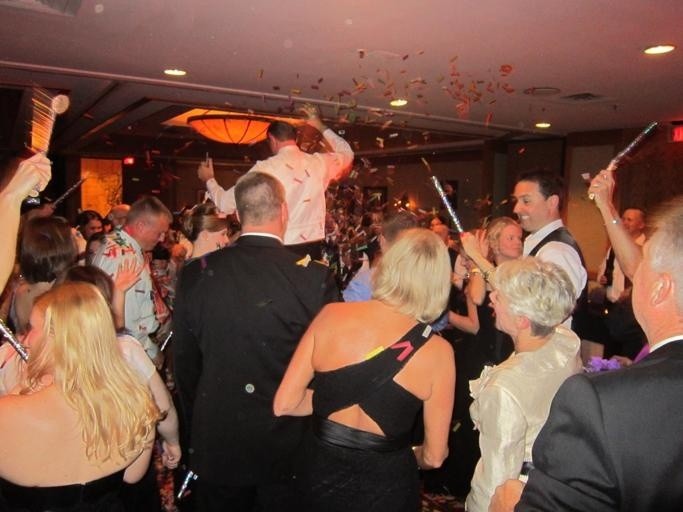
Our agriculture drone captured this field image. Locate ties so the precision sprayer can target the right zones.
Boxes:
[143,251,170,324]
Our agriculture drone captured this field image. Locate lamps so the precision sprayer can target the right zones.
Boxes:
[160,109,308,145]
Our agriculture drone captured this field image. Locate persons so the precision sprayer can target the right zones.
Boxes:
[0,101,682,512]
